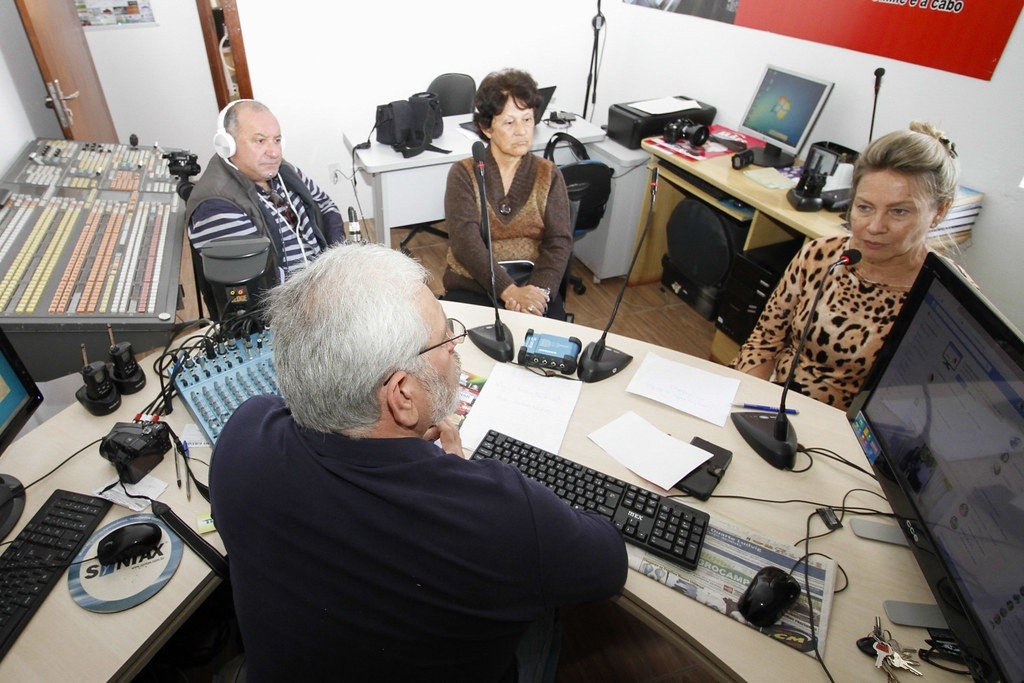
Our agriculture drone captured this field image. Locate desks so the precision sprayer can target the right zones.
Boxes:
[0,111,977,683]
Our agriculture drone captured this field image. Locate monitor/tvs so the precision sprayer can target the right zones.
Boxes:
[847,249,1024,683]
[0,325,43,542]
[738,64,834,169]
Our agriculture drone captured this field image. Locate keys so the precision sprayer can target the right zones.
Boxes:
[856,615,923,683]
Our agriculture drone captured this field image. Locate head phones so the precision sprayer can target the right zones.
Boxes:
[213,98,259,158]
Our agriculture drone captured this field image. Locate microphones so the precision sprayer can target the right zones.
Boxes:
[467,141,514,362]
[732,248,861,471]
[868,66,886,145]
[577,168,660,381]
[224,158,239,170]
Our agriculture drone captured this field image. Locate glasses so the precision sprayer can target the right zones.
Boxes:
[383,317,468,387]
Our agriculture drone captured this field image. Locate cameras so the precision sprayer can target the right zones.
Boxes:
[663,119,709,146]
[99,421,172,484]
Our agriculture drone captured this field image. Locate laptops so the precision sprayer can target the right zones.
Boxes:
[460,86,558,135]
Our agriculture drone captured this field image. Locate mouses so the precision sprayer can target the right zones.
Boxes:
[738,565,801,628]
[97,524,162,566]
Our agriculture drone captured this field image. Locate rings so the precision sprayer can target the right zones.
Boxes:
[528,306,534,312]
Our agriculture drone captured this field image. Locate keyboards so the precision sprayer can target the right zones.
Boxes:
[0,489,114,662]
[469,430,711,569]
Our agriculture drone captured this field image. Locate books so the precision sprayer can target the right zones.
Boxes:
[927,183,984,237]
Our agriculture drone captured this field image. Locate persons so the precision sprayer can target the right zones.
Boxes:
[730,119,978,411]
[208,243,628,683]
[441,68,573,321]
[622,0,738,24]
[185,99,346,284]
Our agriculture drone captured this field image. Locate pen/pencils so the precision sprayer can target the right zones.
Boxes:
[182,440,191,501]
[731,403,801,416]
[173,445,182,488]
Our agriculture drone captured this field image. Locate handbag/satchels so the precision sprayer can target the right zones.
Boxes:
[374,91,452,159]
[497,260,535,287]
